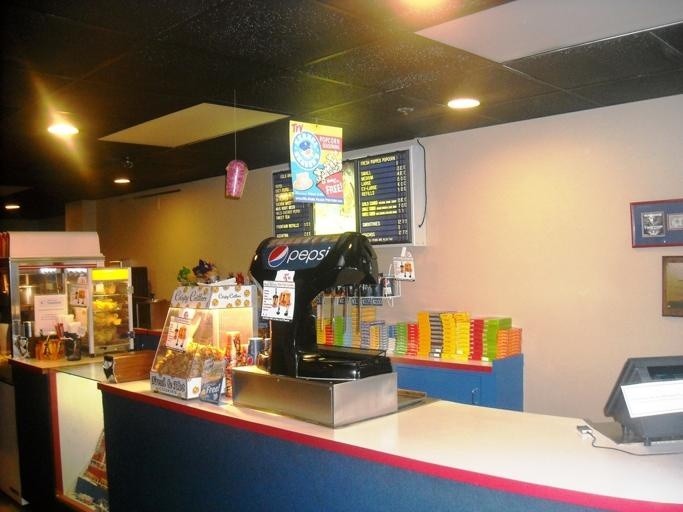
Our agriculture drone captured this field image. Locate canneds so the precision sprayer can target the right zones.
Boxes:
[23,321,32,338]
[12,319,21,335]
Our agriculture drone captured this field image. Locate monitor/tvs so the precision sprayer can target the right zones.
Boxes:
[604,356,682,445]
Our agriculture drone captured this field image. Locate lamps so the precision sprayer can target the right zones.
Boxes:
[224,89,248,200]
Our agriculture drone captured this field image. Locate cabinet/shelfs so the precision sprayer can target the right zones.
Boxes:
[0,231,134,359]
